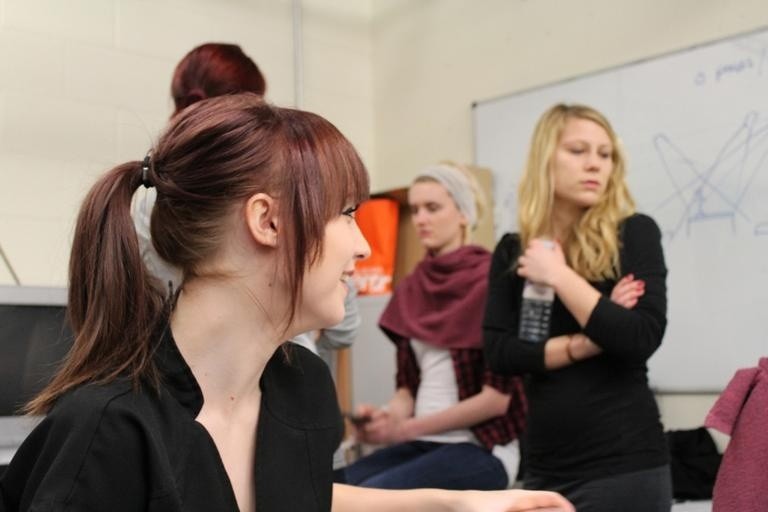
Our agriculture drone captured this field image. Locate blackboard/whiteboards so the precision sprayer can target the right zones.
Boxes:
[471,25,768,394]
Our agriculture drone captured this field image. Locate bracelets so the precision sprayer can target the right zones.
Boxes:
[565,334,578,365]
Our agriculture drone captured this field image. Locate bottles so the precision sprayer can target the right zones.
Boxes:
[519,241,557,342]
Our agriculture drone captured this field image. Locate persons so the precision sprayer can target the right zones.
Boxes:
[0,92,574,512]
[378,159,527,487]
[484,103,676,510]
[305,271,367,454]
[129,42,266,300]
